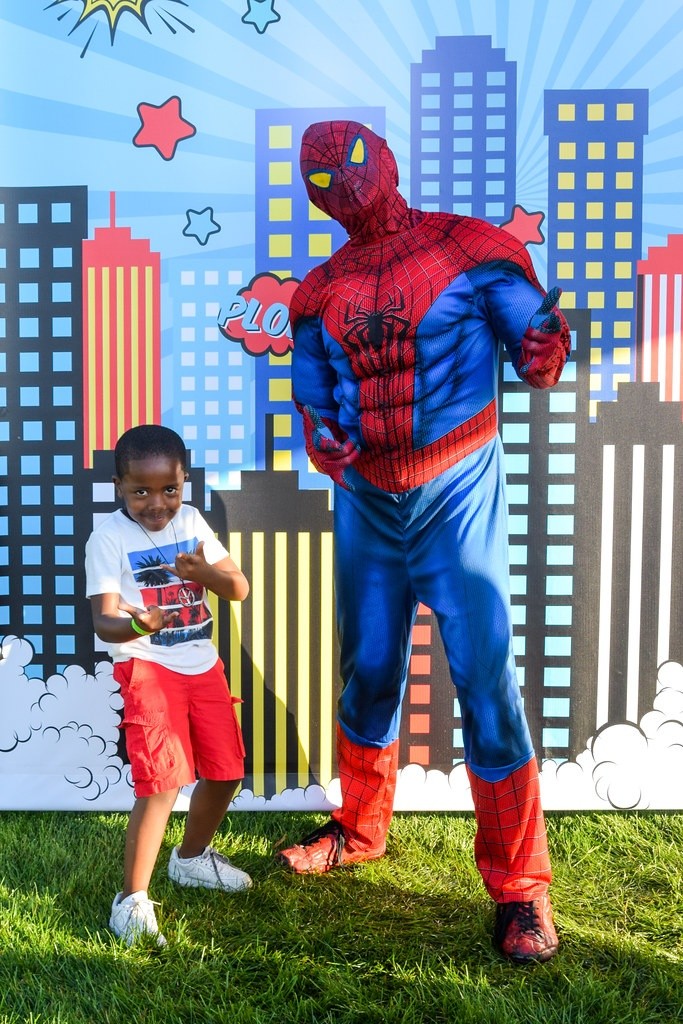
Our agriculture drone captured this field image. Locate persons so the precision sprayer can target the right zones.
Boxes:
[281,120,568,959]
[85,425,254,954]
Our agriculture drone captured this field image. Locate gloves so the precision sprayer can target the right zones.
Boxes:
[520,286,563,379]
[303,403,363,493]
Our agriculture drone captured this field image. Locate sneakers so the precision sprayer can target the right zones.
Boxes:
[167,844,254,892]
[496,893,558,962]
[275,820,387,875]
[109,889,170,955]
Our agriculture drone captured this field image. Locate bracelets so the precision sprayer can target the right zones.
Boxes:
[130,618,156,636]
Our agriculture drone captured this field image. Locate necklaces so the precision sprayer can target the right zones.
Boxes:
[135,520,196,608]
